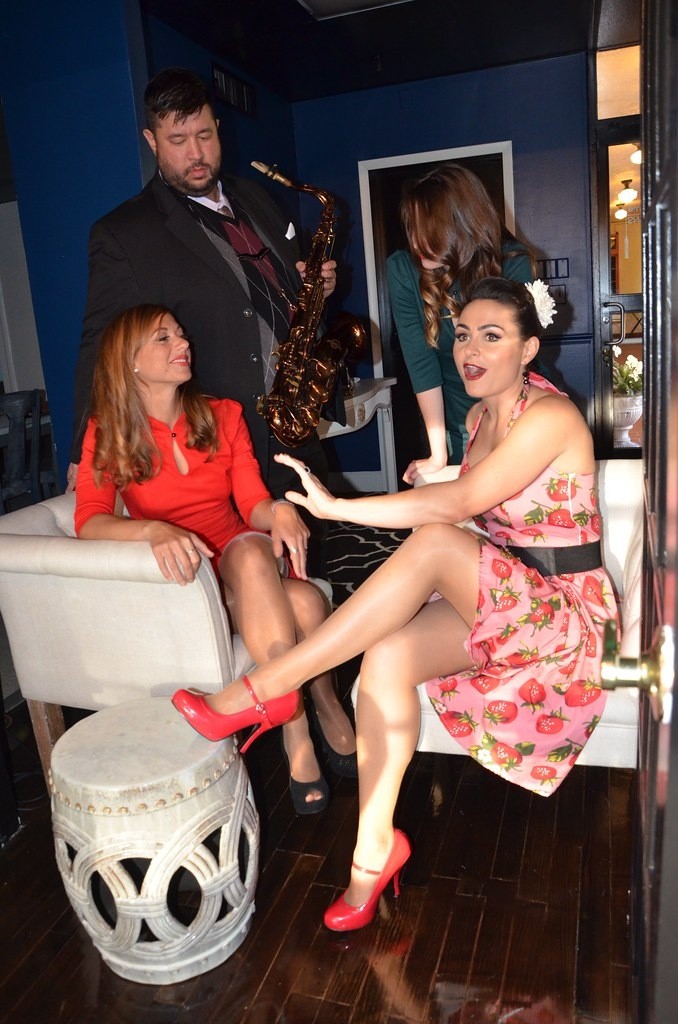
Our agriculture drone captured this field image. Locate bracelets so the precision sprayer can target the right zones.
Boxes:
[271,499,296,514]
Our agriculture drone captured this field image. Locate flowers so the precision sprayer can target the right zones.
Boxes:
[525,277,558,328]
[607,345,643,399]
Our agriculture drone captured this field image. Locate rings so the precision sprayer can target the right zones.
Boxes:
[290,548,298,553]
[412,460,416,463]
[305,547,308,551]
[305,466,311,474]
[188,548,195,553]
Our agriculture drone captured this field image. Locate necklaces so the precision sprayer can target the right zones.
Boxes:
[451,286,461,295]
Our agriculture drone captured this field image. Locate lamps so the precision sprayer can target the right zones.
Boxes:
[613,142,642,221]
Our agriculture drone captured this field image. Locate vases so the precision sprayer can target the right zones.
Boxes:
[610,394,642,448]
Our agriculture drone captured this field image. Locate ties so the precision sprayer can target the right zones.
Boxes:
[217,204,232,219]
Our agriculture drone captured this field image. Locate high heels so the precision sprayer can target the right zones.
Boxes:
[171,675,303,754]
[324,828,411,931]
[280,729,330,814]
[310,702,358,779]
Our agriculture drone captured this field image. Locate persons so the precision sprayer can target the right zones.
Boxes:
[171,277,625,931]
[65,66,337,584]
[385,164,552,486]
[74,305,357,816]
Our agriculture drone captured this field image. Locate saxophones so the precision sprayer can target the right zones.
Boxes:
[251,158,370,451]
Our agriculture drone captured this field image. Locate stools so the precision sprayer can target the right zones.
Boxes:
[48,697,262,986]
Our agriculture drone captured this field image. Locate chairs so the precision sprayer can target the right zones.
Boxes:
[0,387,48,518]
[349,459,647,771]
[0,492,334,804]
